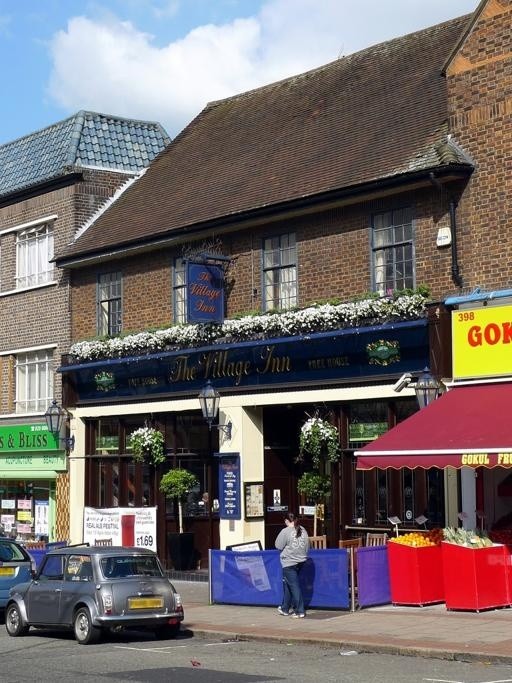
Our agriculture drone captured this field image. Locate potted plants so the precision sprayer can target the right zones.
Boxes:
[159,469,200,571]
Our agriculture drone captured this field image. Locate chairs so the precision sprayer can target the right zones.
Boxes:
[366,533,387,548]
[339,537,364,551]
[308,534,328,550]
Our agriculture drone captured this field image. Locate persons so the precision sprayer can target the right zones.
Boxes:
[276,511,309,618]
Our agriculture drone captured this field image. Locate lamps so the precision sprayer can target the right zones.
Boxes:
[45,399,75,450]
[199,378,232,440]
[414,367,439,409]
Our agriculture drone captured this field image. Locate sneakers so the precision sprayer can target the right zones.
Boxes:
[292,612,305,618]
[278,606,288,616]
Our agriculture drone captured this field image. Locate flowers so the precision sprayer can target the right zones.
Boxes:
[294,413,340,467]
[130,426,165,466]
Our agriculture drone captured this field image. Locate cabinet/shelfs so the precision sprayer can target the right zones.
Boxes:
[439,539,509,614]
[386,539,442,608]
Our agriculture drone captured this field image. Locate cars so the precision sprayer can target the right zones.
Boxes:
[1,540,36,627]
[7,546,184,644]
